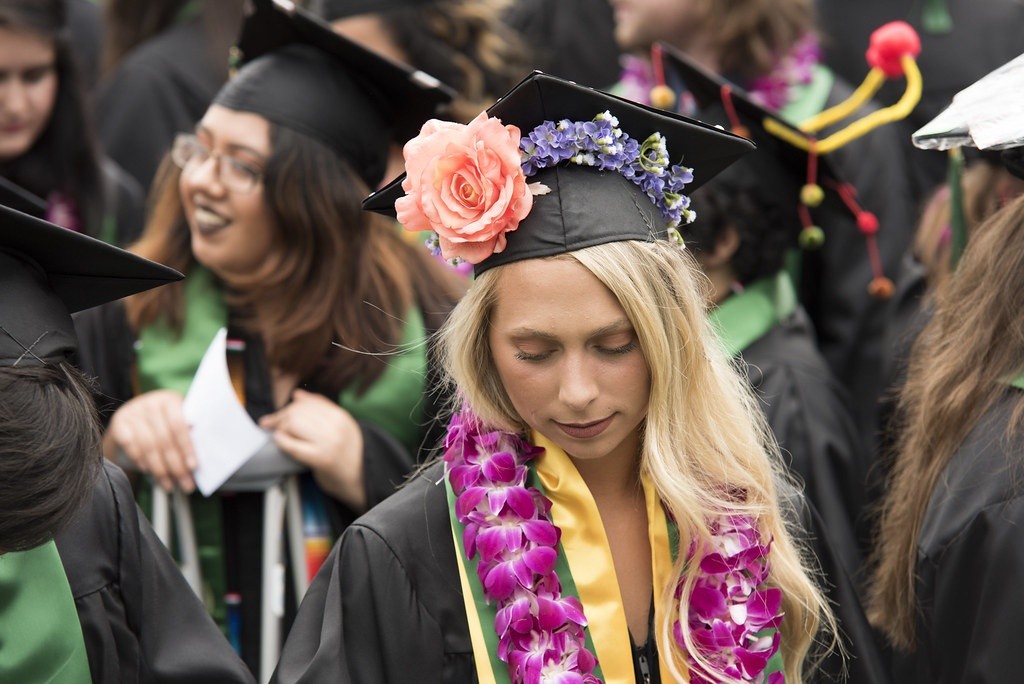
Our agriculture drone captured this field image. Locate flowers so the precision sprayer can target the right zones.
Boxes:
[393,111,695,273]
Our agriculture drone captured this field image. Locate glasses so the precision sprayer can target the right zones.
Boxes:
[172,133,264,192]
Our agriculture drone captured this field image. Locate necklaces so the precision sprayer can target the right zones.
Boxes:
[623,25,817,127]
[441,395,789,684]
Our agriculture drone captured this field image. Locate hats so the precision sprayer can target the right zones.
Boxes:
[912,53,1024,179]
[212,0,460,191]
[648,22,923,301]
[362,70,755,280]
[0,204,183,368]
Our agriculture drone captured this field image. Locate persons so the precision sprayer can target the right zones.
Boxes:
[267,70,848,684]
[603,1,1022,683]
[0,1,528,684]
